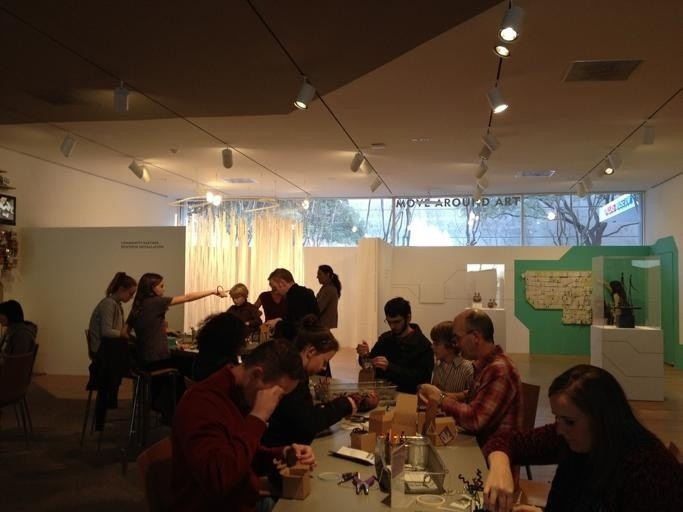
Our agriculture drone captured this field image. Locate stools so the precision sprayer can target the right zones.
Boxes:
[120,364,178,453]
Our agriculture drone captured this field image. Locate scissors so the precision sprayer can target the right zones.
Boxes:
[353,428,361,433]
[314,383,326,403]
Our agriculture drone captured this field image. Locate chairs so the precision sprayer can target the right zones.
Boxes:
[521,382,540,480]
[13,343,38,431]
[519,480,552,509]
[0,352,35,445]
[79,335,140,448]
[135,436,174,512]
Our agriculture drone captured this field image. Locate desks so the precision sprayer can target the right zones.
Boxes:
[170,336,261,380]
[270,393,489,512]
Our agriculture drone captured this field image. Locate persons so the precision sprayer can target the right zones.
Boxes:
[267,315,376,447]
[87,272,137,433]
[168,265,342,409]
[417,310,523,436]
[355,297,434,395]
[120,273,228,415]
[0,298,38,354]
[481,365,681,510]
[428,319,474,394]
[173,338,319,510]
[605,279,628,326]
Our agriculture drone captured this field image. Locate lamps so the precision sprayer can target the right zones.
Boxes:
[0,103,228,193]
[243,0,393,194]
[0,6,310,195]
[472,0,523,203]
[567,88,683,197]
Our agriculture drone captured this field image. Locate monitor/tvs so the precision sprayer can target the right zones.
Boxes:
[0,194,16,226]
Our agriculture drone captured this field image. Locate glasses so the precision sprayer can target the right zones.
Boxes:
[451,329,480,347]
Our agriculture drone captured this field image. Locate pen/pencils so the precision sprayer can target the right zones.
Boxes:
[340,392,347,398]
[379,428,407,465]
[319,372,329,396]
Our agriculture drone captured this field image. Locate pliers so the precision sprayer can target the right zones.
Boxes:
[352,472,376,495]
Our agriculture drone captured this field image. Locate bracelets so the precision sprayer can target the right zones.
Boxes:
[439,392,448,402]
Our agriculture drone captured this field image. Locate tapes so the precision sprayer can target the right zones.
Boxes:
[416,494,445,508]
[318,472,341,482]
